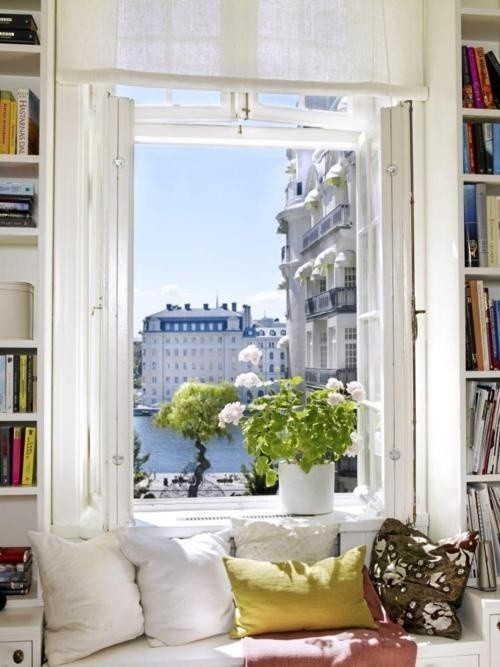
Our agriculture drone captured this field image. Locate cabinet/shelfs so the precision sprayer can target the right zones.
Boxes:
[0,0,56,667]
[454,0,500,667]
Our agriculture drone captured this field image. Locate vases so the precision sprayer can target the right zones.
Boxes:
[277,460,336,516]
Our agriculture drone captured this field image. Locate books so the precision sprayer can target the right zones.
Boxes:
[0,344,39,602]
[459,38,500,599]
[0,11,41,229]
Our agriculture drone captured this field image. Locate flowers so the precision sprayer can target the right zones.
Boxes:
[215,345,363,488]
